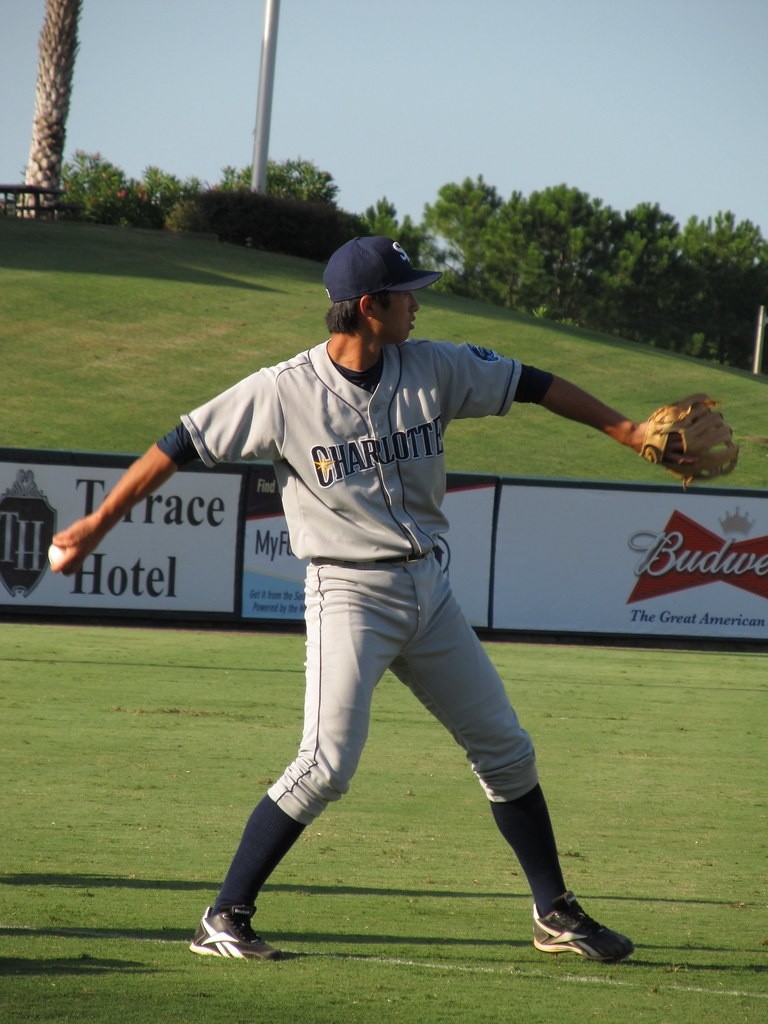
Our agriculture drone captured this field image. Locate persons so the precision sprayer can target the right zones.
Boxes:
[49,236,740,963]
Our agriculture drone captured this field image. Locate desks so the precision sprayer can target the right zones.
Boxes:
[0,183,68,222]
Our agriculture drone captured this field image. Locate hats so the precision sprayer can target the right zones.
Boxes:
[324,235,444,303]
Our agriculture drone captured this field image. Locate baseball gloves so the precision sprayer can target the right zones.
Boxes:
[636,391,740,490]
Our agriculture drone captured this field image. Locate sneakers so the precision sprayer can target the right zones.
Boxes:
[533,890,636,962]
[189,905,283,961]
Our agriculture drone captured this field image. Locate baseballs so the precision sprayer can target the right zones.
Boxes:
[48,542,65,566]
